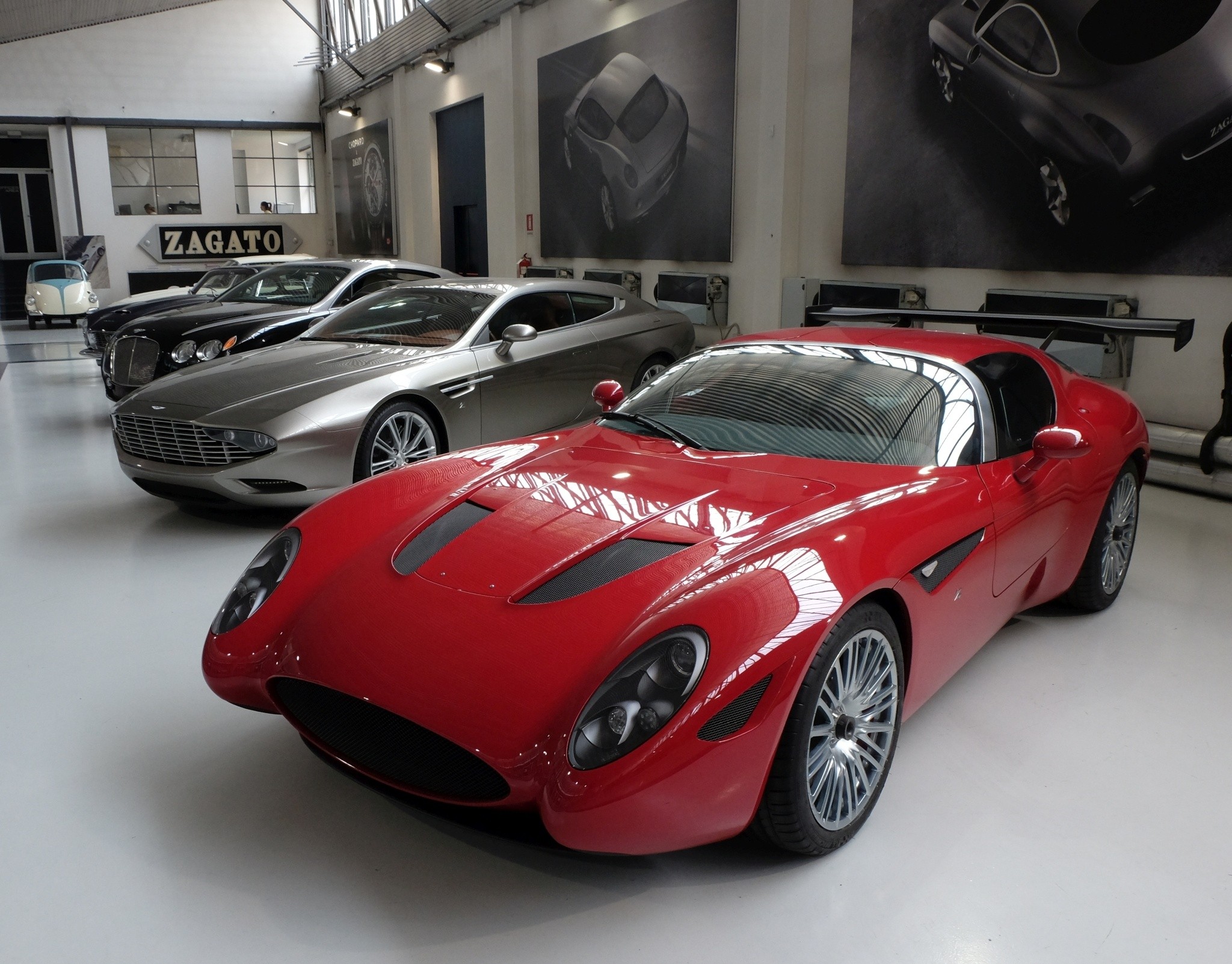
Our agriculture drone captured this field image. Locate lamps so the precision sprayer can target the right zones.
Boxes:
[338,106,361,117]
[424,59,454,74]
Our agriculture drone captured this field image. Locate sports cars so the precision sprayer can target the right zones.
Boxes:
[203,284,1196,856]
[78,252,696,512]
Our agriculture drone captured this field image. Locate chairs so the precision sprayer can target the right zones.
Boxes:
[520,296,559,331]
[312,274,337,293]
[363,274,388,292]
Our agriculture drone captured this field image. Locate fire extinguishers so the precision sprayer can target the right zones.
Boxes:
[519,253,531,278]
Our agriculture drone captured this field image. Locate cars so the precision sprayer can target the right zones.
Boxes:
[24,260,98,328]
[561,52,689,233]
[884,0,1232,250]
